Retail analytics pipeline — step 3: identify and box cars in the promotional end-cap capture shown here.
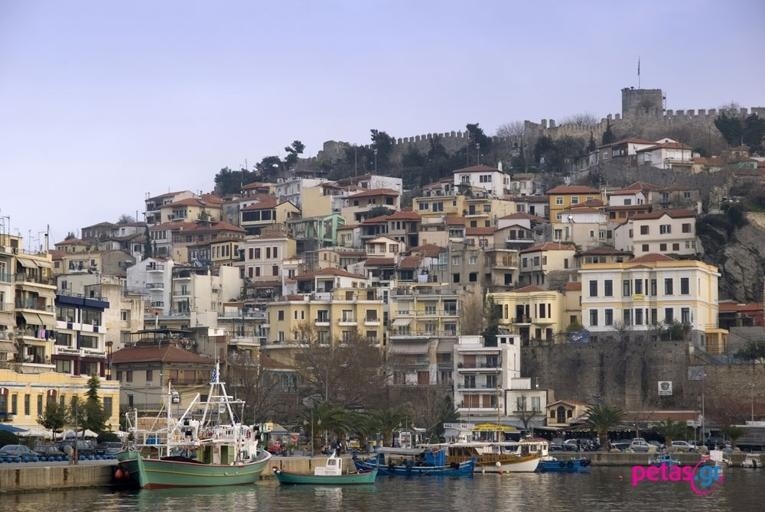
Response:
[1,442,125,457]
[550,438,765,453]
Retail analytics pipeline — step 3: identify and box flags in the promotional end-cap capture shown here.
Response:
[209,365,220,382]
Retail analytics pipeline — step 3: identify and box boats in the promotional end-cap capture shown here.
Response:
[116,362,272,491]
[377,440,591,474]
[352,448,475,480]
[274,458,377,484]
